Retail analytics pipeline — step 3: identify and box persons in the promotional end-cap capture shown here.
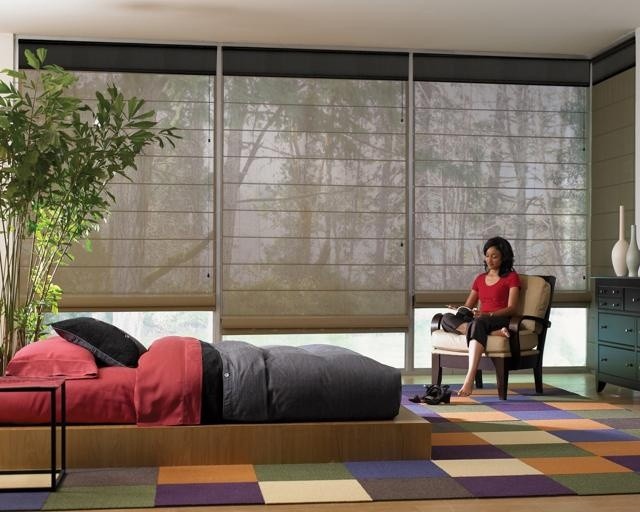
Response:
[458,236,522,398]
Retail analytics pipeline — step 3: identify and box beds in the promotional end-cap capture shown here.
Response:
[0,334,431,470]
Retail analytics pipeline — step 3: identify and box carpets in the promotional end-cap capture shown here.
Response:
[2,382,640,512]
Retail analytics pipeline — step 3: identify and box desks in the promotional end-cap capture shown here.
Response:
[1,376,66,492]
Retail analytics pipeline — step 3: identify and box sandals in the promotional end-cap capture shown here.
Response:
[408,384,452,405]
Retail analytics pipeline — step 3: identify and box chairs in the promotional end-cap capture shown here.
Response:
[431,275,556,400]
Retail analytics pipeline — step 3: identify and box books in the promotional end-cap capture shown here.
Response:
[444,305,474,322]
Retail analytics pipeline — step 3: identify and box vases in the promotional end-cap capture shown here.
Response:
[611,206,640,277]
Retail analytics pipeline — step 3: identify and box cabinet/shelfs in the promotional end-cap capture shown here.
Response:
[590,277,640,393]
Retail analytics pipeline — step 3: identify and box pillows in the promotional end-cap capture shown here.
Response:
[5,318,147,380]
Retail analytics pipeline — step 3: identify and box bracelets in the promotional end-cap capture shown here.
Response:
[489,312,494,318]
